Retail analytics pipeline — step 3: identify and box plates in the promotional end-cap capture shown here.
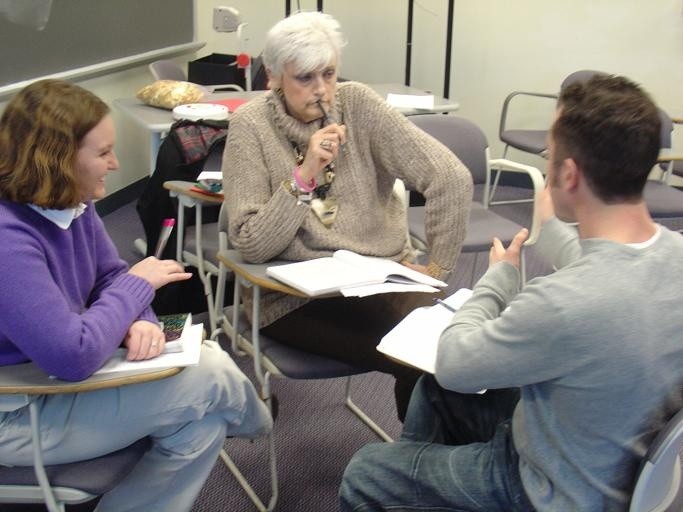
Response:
[172,103,229,122]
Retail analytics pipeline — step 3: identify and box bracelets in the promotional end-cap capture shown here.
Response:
[293,164,316,194]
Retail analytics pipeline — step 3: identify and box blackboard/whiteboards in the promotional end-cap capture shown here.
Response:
[0,0,205,99]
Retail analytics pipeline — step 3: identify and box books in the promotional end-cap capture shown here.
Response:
[266,250,450,298]
[47,313,203,377]
[377,288,489,397]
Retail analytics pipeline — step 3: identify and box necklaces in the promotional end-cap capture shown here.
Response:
[264,83,347,229]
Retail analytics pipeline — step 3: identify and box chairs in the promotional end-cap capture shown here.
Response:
[252,51,271,90]
[490,71,683,234]
[150,60,244,94]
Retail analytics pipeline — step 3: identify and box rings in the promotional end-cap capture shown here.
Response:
[149,341,158,347]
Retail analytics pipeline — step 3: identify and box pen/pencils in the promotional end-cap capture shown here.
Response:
[318,101,330,124]
[433,297,457,313]
[153,218,175,259]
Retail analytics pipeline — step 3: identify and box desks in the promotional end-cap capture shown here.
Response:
[112,83,459,260]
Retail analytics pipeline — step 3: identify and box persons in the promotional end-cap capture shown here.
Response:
[336,73,683,512]
[0,81,275,512]
[217,10,474,426]
